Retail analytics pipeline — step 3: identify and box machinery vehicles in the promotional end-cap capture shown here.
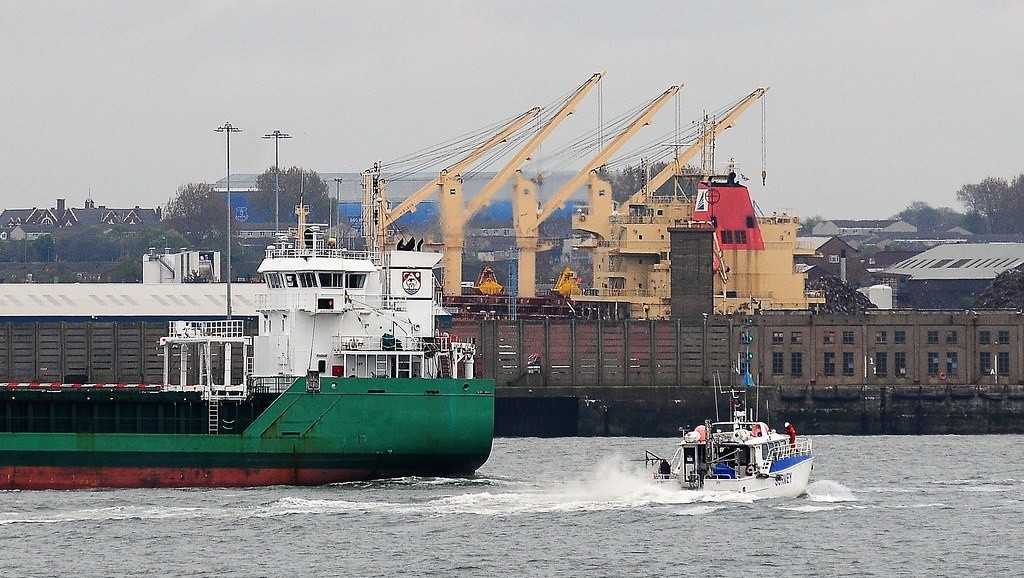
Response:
[362,69,769,302]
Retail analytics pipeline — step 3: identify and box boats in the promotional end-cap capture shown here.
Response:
[636,371,815,502]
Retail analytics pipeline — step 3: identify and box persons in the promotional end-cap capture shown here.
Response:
[784,422,796,448]
[659,460,671,479]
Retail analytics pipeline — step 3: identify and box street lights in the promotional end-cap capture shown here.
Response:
[334,178,344,247]
[261,130,293,248]
[213,122,242,322]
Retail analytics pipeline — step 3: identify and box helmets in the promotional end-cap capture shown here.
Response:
[784,422,790,428]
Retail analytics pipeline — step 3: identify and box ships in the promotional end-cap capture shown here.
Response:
[0,235,497,494]
